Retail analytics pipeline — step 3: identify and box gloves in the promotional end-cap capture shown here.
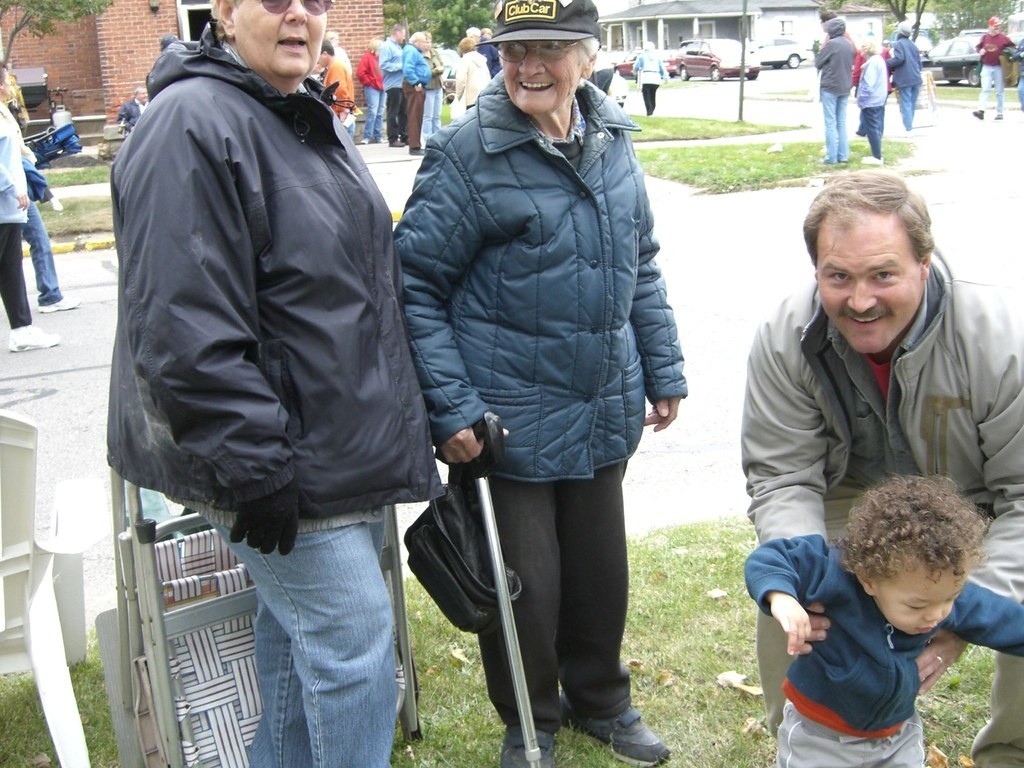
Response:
[229,471,300,557]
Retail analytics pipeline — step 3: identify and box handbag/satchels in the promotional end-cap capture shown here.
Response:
[404,482,522,633]
[441,88,467,126]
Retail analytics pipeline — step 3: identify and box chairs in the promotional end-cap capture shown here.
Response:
[0,412,93,768]
[98,437,422,768]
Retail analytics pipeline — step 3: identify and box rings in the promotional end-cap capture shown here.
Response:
[937,655,944,664]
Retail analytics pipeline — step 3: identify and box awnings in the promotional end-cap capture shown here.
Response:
[8,67,49,88]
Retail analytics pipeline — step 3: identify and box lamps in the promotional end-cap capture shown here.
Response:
[150,0,159,14]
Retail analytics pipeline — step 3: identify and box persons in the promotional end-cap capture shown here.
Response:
[852,27,893,137]
[886,24,922,138]
[819,10,858,63]
[854,41,888,165]
[735,171,1024,767]
[972,15,1014,121]
[311,24,624,154]
[1013,37,1024,113]
[744,471,1024,767]
[0,63,82,352]
[393,0,689,767]
[115,82,151,136]
[814,15,853,164]
[106,0,447,768]
[632,39,669,120]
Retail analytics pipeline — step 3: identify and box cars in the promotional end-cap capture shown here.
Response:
[675,36,760,82]
[751,37,809,70]
[885,28,1024,89]
[615,47,679,81]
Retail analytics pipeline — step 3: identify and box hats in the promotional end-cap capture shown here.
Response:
[475,0,601,60]
[989,16,1002,28]
[898,21,911,37]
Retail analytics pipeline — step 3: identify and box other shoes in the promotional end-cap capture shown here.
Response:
[856,131,867,136]
[360,138,370,144]
[861,155,884,165]
[819,157,848,166]
[376,139,388,144]
[389,141,405,147]
[975,742,1024,768]
[995,114,1003,120]
[973,109,984,120]
[50,197,63,211]
[409,146,425,155]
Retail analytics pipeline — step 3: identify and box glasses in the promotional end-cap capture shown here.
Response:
[493,39,584,62]
[261,0,335,16]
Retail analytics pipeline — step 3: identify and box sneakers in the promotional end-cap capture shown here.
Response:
[9,327,59,352]
[38,292,80,312]
[559,687,670,768]
[498,721,556,768]
